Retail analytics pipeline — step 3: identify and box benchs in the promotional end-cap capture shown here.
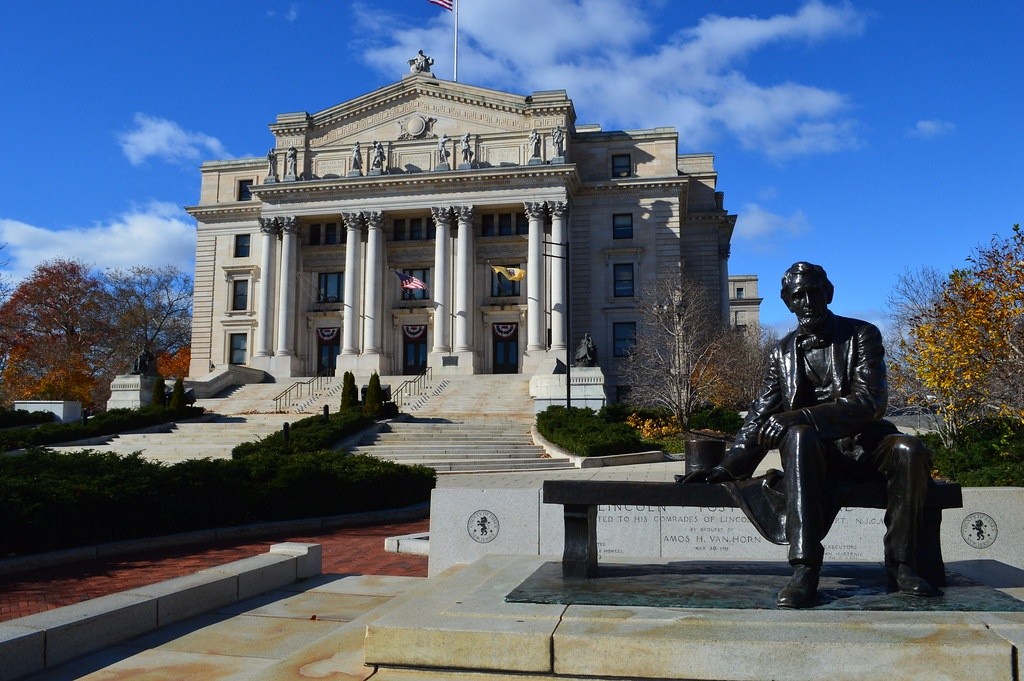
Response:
[543,479,963,586]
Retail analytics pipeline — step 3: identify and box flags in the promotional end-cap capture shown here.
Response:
[429,0,453,11]
[392,270,428,289]
[491,266,526,281]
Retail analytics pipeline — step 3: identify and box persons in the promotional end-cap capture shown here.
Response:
[406,49,430,73]
[129,347,156,376]
[677,263,936,607]
[577,332,593,360]
[265,125,565,177]
